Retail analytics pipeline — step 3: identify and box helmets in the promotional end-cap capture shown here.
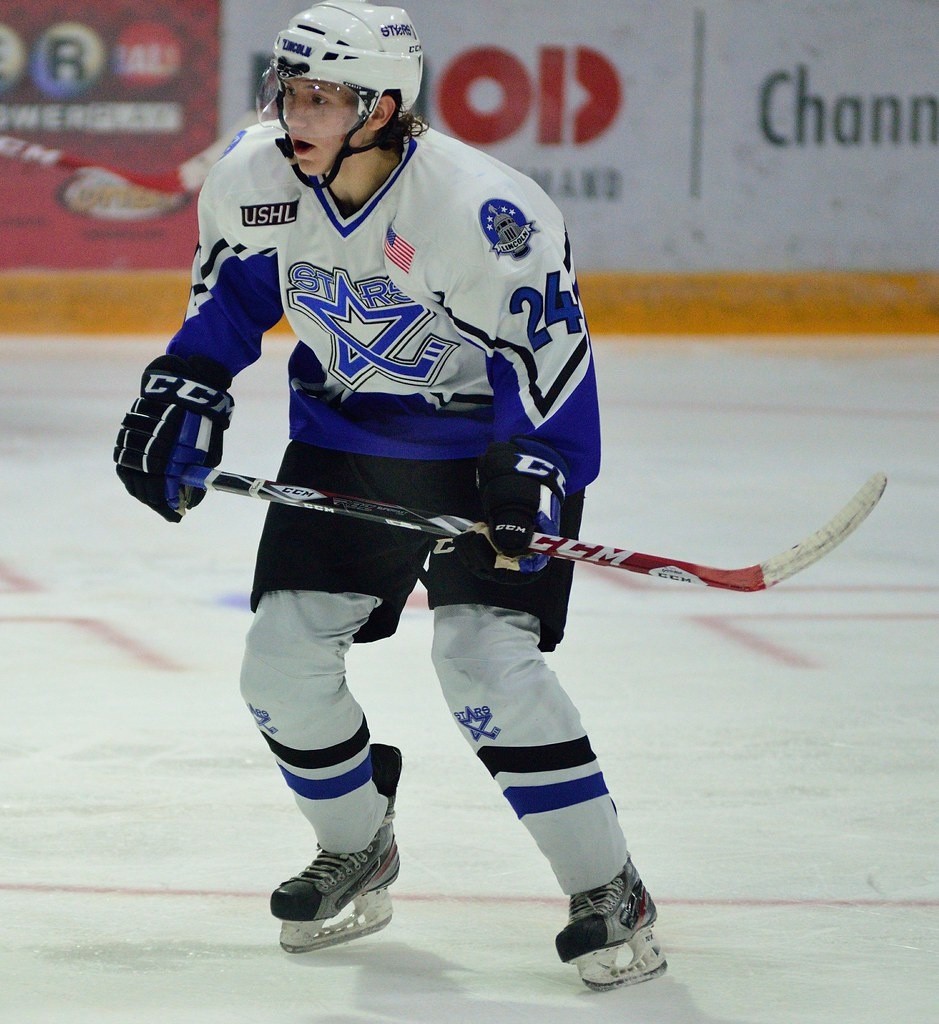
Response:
[272,0,424,123]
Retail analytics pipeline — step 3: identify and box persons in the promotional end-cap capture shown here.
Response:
[113,1,668,993]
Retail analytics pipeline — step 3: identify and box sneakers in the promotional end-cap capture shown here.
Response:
[269,791,403,954]
[555,851,667,993]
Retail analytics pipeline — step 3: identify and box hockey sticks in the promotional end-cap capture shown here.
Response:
[171,466,892,596]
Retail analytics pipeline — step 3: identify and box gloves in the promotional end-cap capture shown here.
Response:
[452,454,568,577]
[114,354,236,524]
